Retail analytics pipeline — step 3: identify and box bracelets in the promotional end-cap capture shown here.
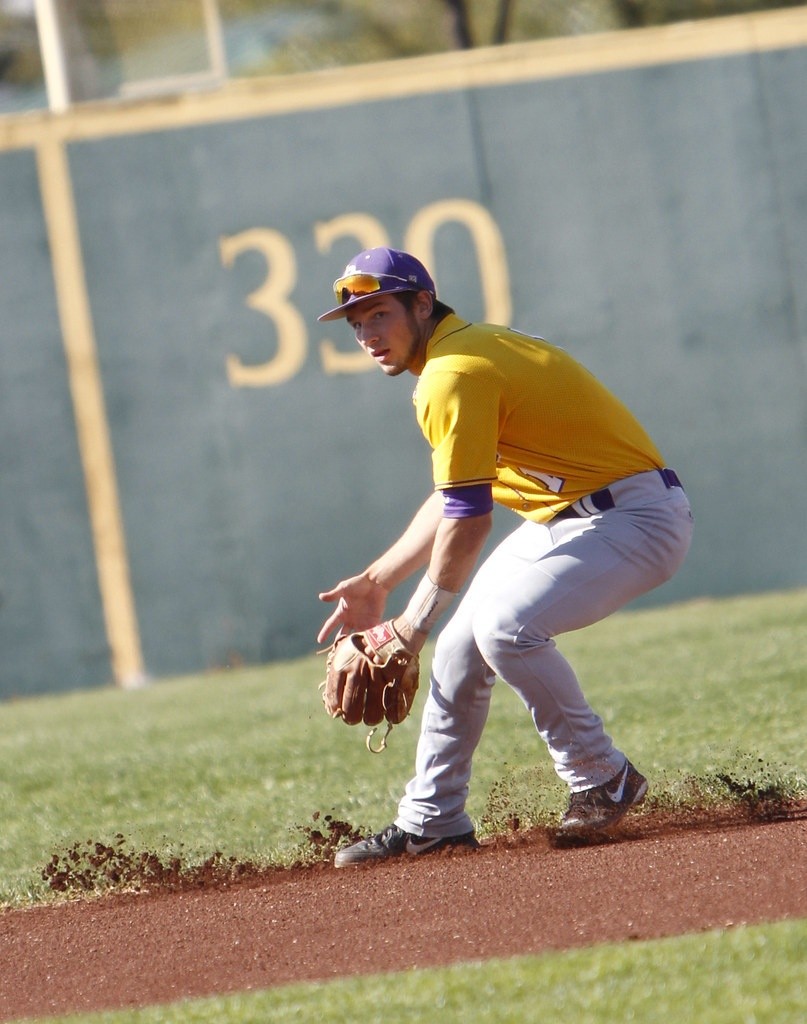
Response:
[403,571,461,634]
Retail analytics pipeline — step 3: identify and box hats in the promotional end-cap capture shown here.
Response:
[317,247,436,321]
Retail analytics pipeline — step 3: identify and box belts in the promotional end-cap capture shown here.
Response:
[555,469,682,518]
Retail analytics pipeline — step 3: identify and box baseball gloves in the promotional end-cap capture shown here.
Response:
[316,617,421,755]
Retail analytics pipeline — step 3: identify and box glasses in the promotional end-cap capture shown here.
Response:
[332,272,434,306]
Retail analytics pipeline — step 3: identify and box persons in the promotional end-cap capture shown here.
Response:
[317,247,694,870]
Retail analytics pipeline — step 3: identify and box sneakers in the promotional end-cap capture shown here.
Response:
[561,758,648,837]
[334,824,480,868]
